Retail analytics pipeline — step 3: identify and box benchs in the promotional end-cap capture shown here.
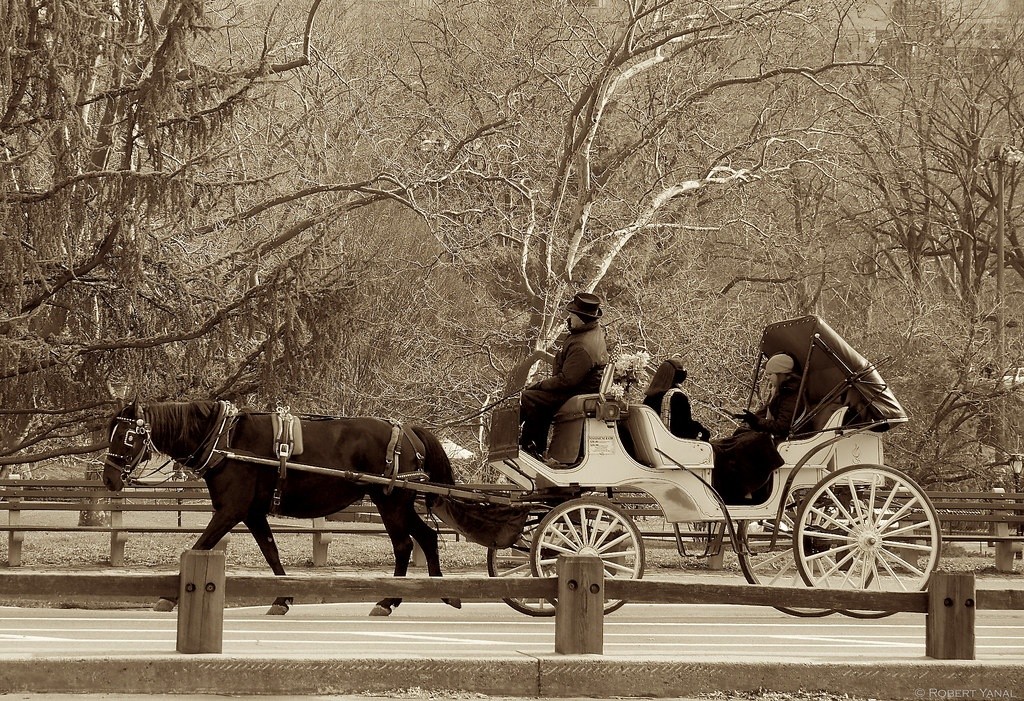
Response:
[628,402,714,469]
[777,402,849,470]
[546,341,620,463]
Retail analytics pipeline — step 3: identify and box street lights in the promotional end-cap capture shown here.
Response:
[974,144,1024,489]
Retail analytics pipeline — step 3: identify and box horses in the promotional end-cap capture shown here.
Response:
[102,393,462,616]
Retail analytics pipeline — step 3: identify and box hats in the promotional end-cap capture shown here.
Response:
[766,354,794,373]
[567,294,602,318]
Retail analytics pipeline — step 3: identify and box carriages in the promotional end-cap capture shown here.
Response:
[103,315,941,619]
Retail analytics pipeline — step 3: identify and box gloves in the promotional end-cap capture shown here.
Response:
[735,409,758,425]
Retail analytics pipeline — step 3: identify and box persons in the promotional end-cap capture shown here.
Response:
[643,360,709,440]
[731,352,809,498]
[517,291,609,460]
